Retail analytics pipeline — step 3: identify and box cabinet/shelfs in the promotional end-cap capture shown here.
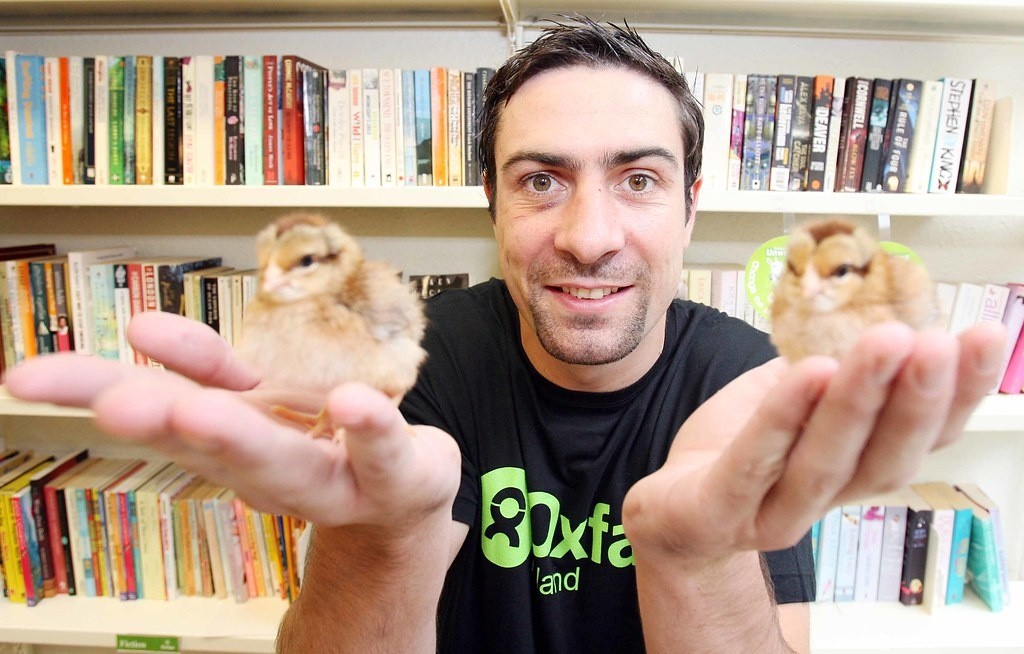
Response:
[0,0,1024,654]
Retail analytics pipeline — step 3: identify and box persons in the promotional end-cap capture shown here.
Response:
[0,21,1009,654]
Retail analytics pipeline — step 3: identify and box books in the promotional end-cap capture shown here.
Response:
[674,265,1024,394]
[0,448,313,607]
[0,50,497,188]
[0,243,470,384]
[664,57,1015,196]
[811,483,1010,612]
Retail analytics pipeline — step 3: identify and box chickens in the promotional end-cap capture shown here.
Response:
[767,220,946,369]
[232,211,428,446]
